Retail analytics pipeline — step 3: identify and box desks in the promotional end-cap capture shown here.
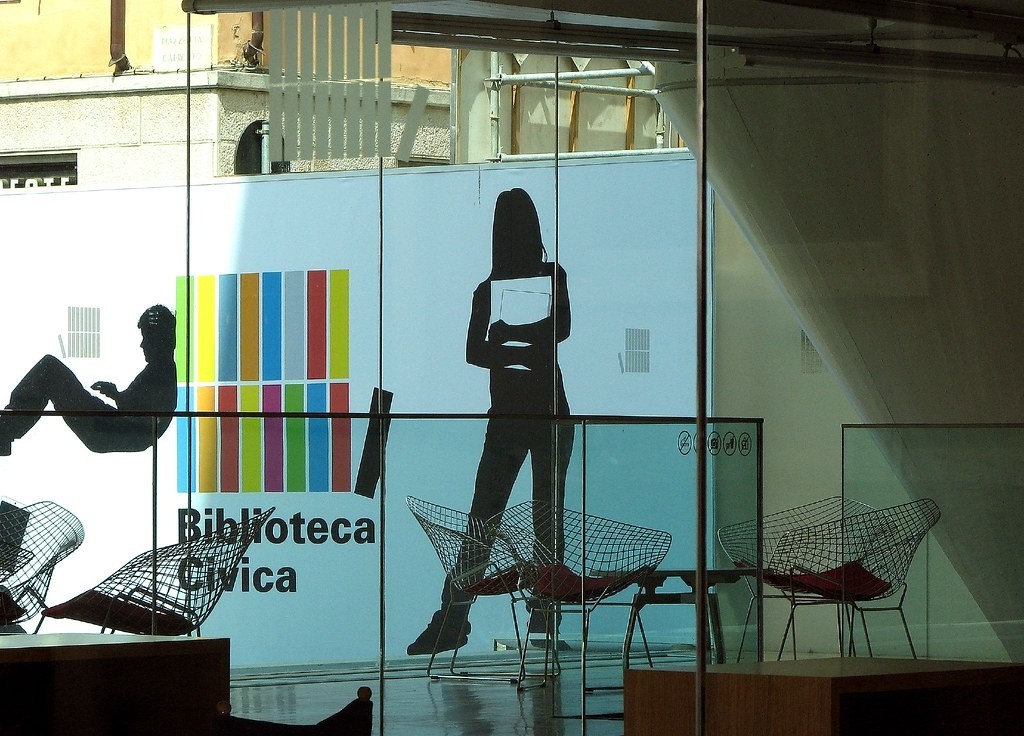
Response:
[589,568,772,671]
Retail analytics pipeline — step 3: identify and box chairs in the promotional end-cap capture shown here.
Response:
[41,506,276,637]
[405,494,563,684]
[0,500,86,635]
[716,493,942,664]
[483,499,672,692]
